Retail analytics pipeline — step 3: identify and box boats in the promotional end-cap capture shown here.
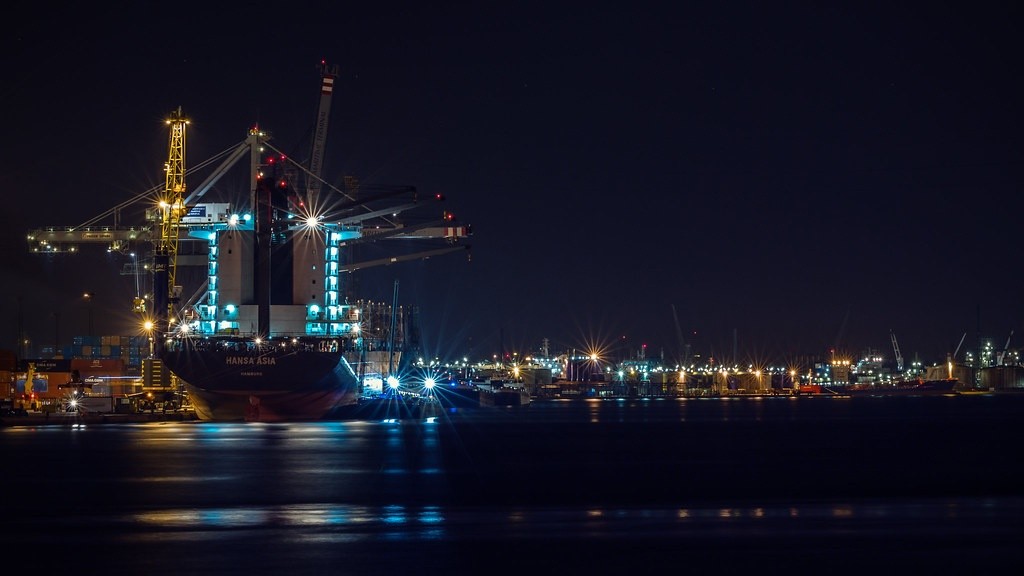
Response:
[496,388,531,413]
[825,377,958,398]
